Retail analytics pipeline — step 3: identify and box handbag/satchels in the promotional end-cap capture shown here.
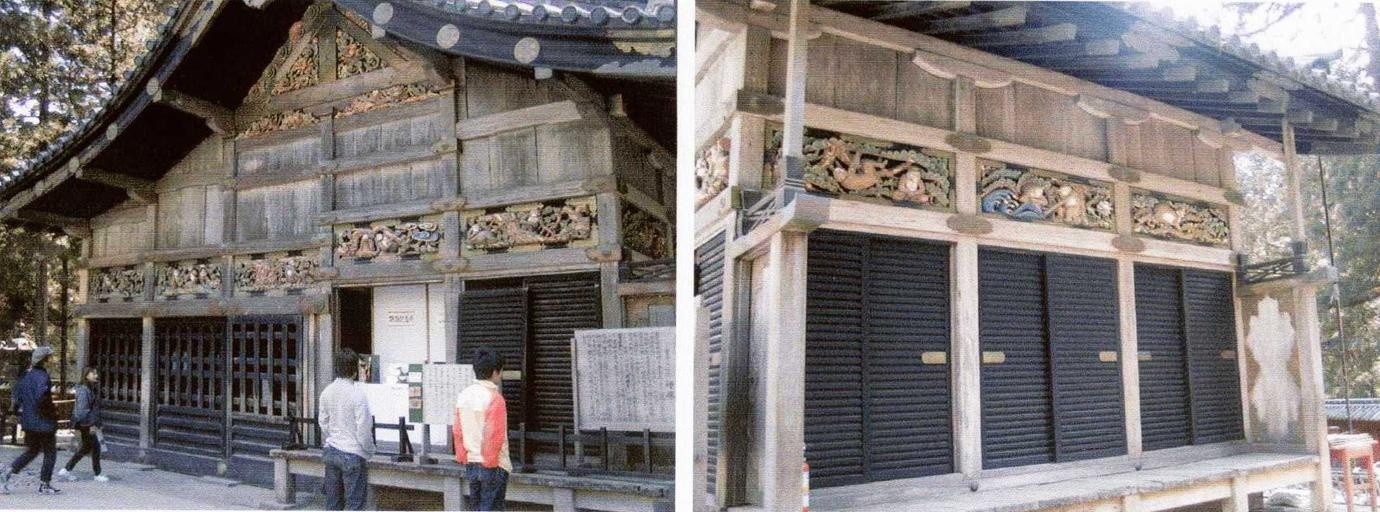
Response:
[70,415,80,429]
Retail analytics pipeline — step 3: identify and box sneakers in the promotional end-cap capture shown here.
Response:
[39,484,61,494]
[94,475,109,481]
[59,468,77,481]
[0,467,11,493]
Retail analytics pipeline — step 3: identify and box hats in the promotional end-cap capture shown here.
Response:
[32,348,51,364]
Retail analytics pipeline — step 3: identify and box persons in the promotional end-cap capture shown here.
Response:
[318,346,376,510]
[56,368,110,482]
[0,346,62,495]
[452,345,512,511]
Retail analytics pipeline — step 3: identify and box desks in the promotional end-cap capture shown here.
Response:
[1328,442,1378,512]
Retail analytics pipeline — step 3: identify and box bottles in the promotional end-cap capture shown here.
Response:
[96,429,108,453]
